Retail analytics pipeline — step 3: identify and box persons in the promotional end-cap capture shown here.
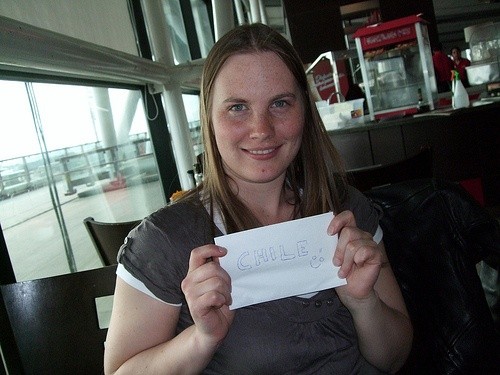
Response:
[104,23,413,375]
[432,46,471,94]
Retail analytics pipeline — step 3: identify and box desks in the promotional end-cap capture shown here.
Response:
[327,97,500,167]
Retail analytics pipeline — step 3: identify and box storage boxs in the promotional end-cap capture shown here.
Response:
[315,98,366,132]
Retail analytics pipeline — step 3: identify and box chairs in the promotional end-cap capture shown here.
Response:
[0,264,118,375]
[83,217,141,266]
[340,143,434,192]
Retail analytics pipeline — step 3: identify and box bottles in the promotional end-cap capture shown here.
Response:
[417,88,425,113]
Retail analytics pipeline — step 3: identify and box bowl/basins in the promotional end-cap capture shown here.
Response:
[466,61,499,86]
